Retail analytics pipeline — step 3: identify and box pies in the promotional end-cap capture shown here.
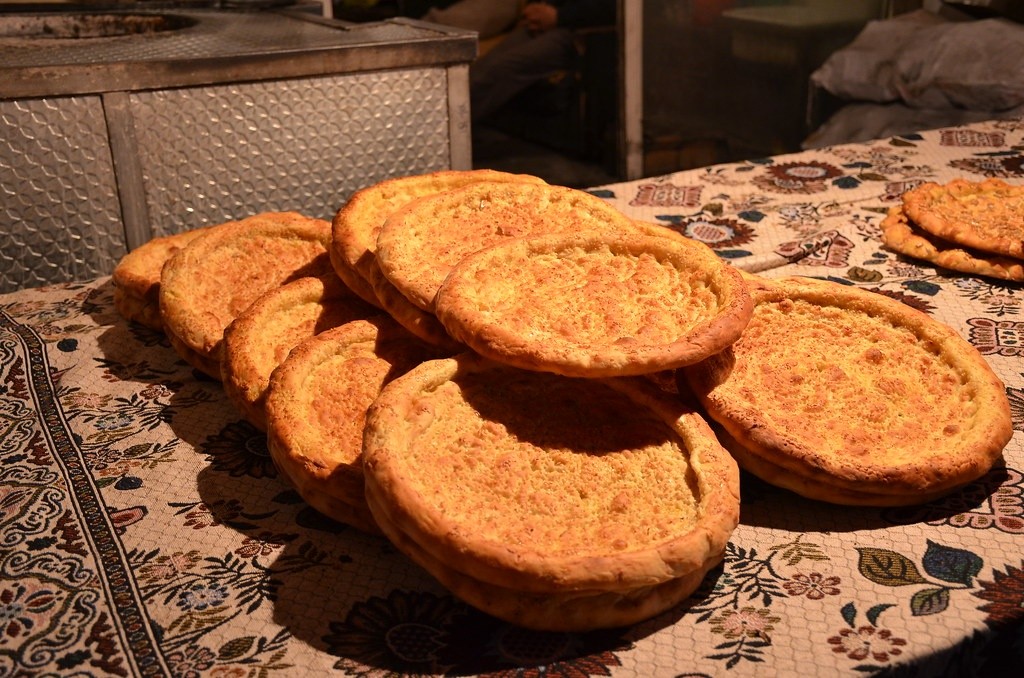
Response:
[111,169,1024,636]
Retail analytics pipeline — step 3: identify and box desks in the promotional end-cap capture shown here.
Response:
[0,117,1024,678]
